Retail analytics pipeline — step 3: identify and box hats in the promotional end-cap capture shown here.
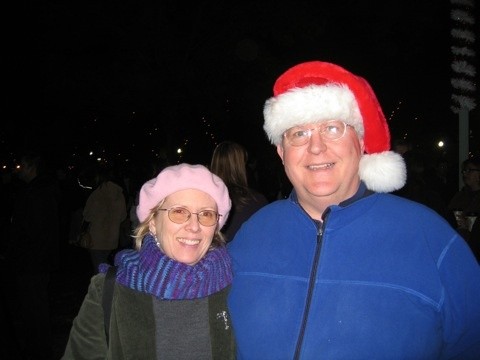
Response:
[135,163,232,231]
[263,61,406,193]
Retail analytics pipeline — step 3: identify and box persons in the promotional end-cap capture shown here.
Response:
[227,61,480,360]
[62,164,238,360]
[0,135,480,360]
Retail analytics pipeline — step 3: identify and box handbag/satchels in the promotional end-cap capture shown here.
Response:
[76,217,92,248]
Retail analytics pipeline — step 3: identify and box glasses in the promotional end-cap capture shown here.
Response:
[158,206,221,227]
[462,168,480,175]
[282,120,354,147]
